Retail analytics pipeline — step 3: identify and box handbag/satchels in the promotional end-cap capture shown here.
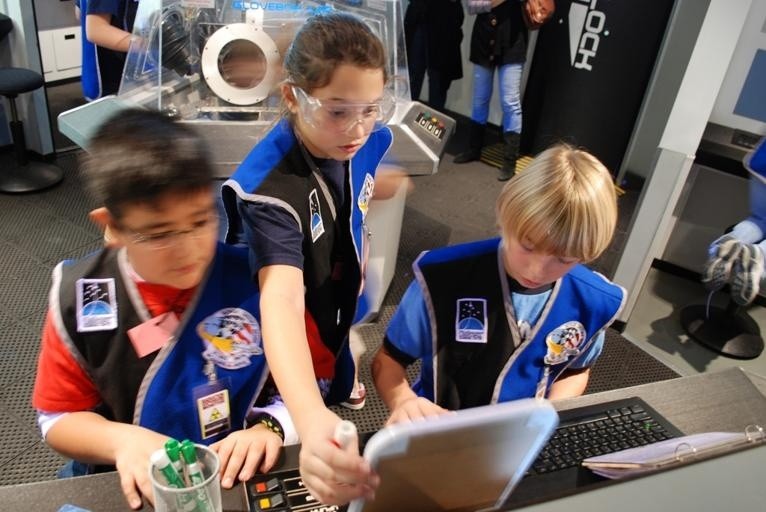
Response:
[521,0,556,29]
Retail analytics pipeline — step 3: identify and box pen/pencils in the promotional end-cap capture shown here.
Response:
[150,438,217,512]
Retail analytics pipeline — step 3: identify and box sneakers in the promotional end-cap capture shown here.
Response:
[701,234,762,307]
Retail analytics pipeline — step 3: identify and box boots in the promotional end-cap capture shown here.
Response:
[453,121,487,162]
[498,131,521,182]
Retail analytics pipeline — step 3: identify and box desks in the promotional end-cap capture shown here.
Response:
[1,367,766,512]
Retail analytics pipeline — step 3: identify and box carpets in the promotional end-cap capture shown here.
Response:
[480,133,629,199]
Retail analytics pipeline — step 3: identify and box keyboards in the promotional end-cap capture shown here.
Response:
[500,397,687,512]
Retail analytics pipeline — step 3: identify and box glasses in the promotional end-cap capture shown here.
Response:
[114,213,220,250]
[292,86,398,136]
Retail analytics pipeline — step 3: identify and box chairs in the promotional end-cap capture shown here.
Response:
[0,12,68,193]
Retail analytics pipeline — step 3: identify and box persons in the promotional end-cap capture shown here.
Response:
[370,143,631,429]
[223,11,393,509]
[403,1,467,112]
[452,2,532,179]
[702,140,766,304]
[75,1,153,102]
[31,107,338,512]
